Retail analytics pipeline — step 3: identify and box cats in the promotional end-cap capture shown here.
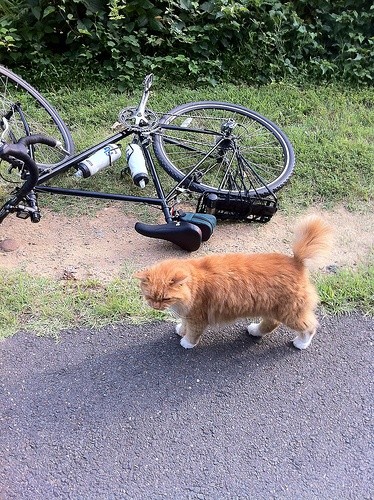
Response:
[133,215,330,350]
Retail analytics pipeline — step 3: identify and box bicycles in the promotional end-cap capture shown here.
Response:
[1,68,295,251]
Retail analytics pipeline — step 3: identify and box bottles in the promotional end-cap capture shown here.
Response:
[126,143,150,191]
[75,143,123,180]
